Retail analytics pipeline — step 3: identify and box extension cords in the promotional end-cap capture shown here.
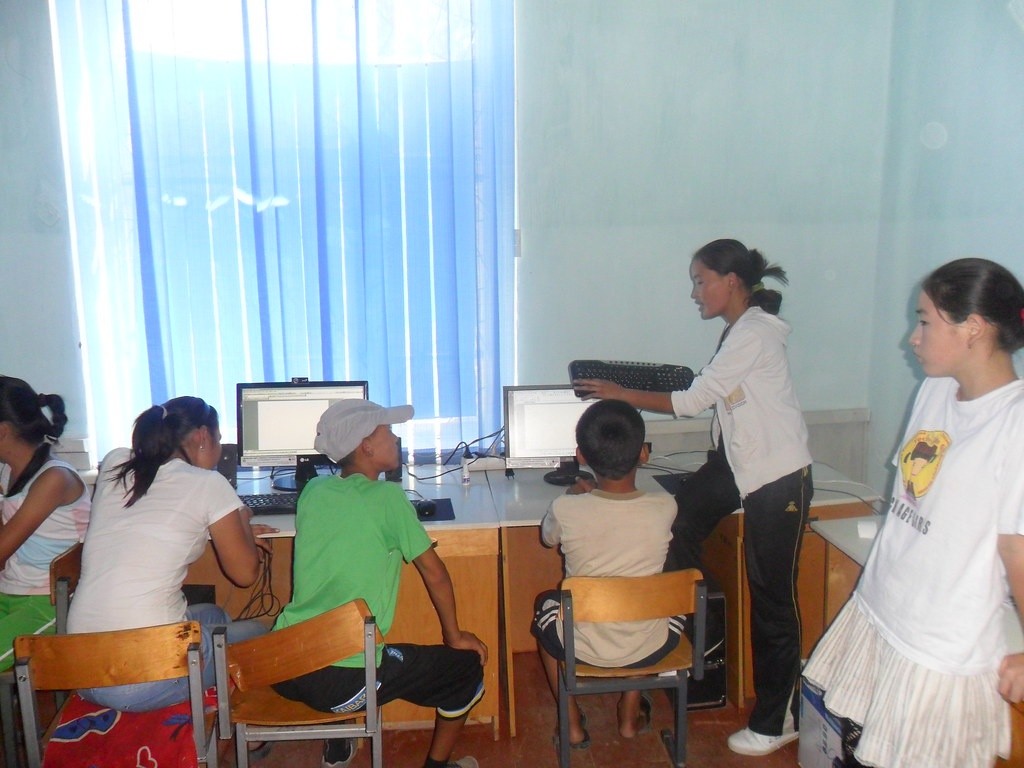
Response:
[460,455,506,471]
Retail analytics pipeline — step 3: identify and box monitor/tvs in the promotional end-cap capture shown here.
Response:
[236,380,369,491]
[503,384,594,485]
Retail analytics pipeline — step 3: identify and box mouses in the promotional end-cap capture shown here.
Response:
[417,500,436,518]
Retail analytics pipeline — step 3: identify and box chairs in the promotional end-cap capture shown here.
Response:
[555,568,707,768]
[0,540,383,768]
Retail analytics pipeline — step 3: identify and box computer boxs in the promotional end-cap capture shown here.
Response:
[670,579,728,709]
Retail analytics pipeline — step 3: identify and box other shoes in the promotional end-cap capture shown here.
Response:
[447,755,479,768]
[321,737,359,768]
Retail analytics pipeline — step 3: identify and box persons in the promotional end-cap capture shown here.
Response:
[0,374,92,673]
[801,258,1024,768]
[269,398,489,768]
[572,238,815,756]
[65,396,280,759]
[529,400,688,751]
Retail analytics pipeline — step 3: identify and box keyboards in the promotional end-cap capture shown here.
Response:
[238,493,299,514]
[569,359,694,398]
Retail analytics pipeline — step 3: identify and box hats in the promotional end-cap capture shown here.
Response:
[314,398,414,463]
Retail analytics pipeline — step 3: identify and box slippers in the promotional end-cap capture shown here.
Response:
[637,693,654,737]
[569,701,592,751]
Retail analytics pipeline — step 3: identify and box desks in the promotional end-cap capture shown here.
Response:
[811,514,1024,768]
[484,454,882,740]
[182,464,500,742]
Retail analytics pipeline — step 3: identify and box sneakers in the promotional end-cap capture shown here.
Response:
[726,714,799,756]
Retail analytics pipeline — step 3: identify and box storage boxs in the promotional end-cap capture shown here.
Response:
[798,659,842,768]
